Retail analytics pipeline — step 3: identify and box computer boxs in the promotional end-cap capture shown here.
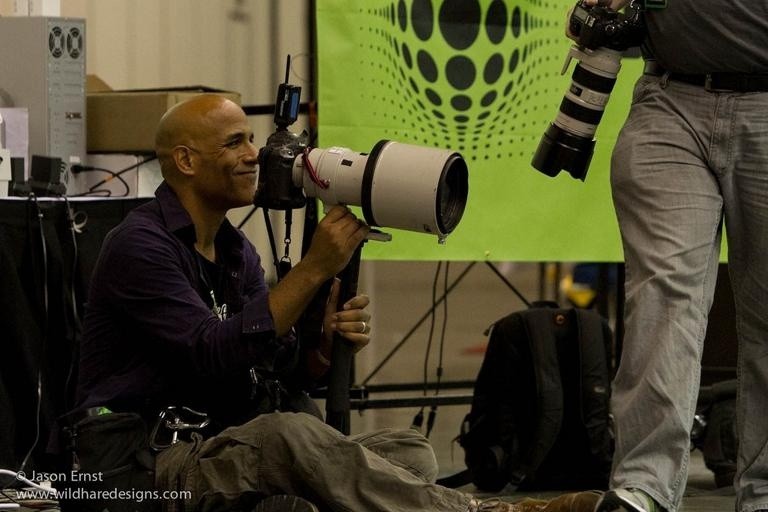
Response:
[0,16,88,197]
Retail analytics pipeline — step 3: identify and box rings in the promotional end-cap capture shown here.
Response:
[360,321,366,333]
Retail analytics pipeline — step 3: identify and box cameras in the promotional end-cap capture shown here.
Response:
[531,0,642,183]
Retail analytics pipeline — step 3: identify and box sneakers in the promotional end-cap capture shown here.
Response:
[252,495,318,512]
[594,487,668,512]
[466,490,603,512]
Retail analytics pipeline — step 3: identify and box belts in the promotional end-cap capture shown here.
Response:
[643,58,768,93]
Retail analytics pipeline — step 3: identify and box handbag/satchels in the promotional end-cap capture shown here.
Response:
[62,413,148,478]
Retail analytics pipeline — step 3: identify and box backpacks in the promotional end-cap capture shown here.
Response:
[460,301,615,492]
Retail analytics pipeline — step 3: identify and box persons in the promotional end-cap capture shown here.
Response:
[67,93,619,512]
[576,1,768,512]
